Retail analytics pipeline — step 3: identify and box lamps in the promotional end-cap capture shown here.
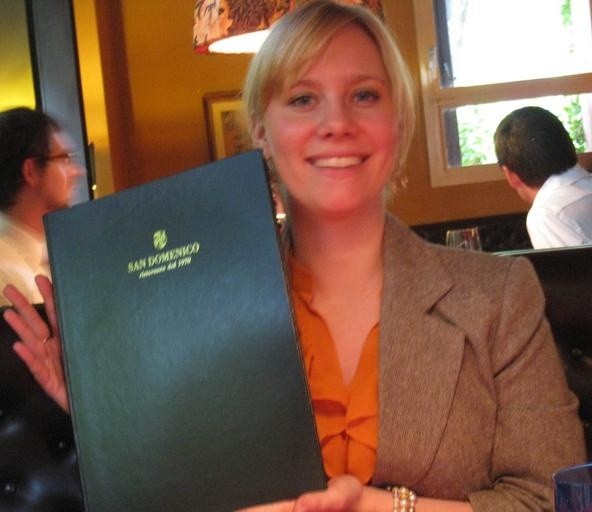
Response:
[192,1,384,57]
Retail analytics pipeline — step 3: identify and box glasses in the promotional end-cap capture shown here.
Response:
[49,150,73,161]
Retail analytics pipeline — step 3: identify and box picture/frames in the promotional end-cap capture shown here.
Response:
[202,89,288,225]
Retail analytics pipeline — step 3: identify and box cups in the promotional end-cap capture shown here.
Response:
[552,462,592,511]
[444,229,481,251]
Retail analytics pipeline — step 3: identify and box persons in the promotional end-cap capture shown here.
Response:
[493,104,591,250]
[0,106,82,306]
[3,0,588,512]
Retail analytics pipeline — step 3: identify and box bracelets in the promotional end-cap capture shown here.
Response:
[387,482,418,512]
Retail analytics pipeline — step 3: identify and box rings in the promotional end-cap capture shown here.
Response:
[42,335,52,343]
